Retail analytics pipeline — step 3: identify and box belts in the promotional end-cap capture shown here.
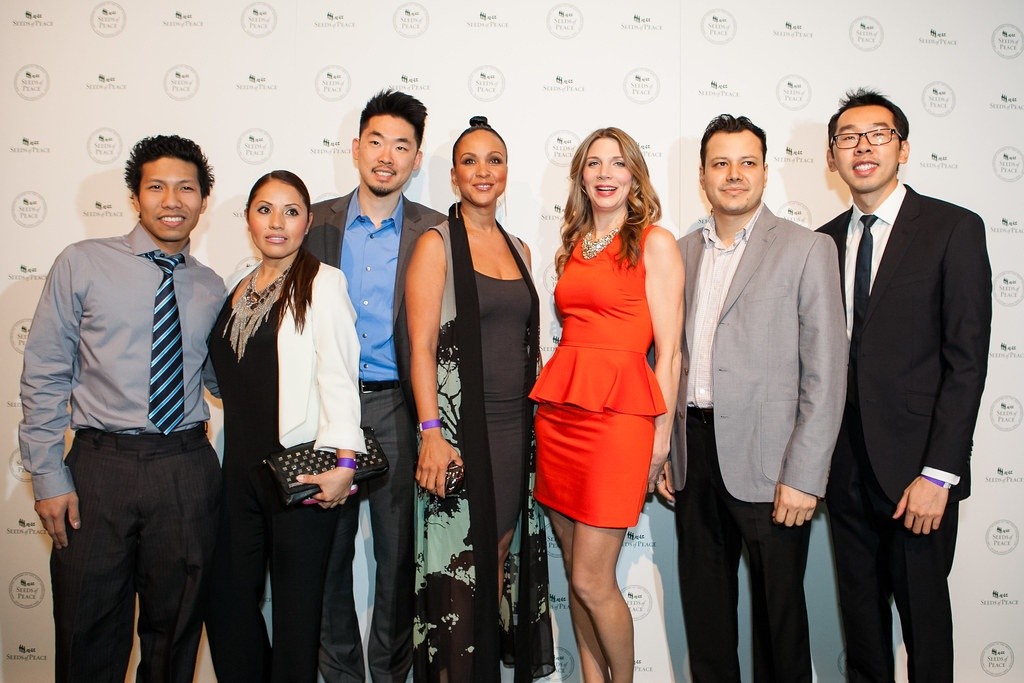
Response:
[687,408,714,424]
[359,380,400,394]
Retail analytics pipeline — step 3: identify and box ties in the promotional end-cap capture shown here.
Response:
[848,214,877,398]
[138,251,185,436]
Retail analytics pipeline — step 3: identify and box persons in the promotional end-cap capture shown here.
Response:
[403,116,545,683]
[811,88,992,683]
[18,134,229,683]
[528,127,685,683]
[646,113,849,683]
[204,170,368,683]
[306,88,449,683]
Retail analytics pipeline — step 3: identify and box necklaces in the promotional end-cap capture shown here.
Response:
[581,227,620,261]
[222,263,294,364]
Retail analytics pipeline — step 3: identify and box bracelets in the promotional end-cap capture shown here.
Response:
[418,419,442,432]
[920,475,951,489]
[336,458,359,470]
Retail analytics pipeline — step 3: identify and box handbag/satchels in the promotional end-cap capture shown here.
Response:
[261,426,388,505]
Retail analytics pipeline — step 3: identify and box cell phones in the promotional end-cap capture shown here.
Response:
[301,483,359,504]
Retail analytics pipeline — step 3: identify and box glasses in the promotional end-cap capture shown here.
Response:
[829,128,905,150]
